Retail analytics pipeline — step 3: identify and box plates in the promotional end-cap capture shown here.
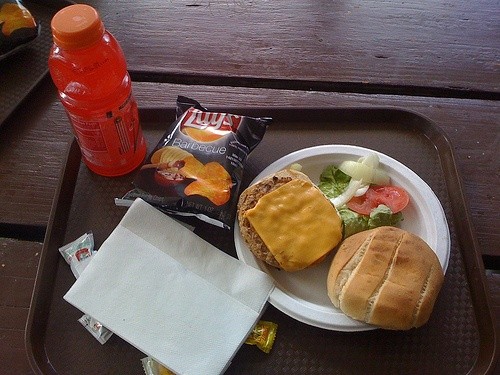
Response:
[234,144,451,332]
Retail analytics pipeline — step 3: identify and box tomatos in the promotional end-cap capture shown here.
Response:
[346,183,409,216]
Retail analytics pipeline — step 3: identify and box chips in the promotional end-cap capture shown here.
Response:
[0,2,37,36]
[150,146,233,206]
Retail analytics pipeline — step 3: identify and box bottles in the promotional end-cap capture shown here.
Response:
[48,4,148,179]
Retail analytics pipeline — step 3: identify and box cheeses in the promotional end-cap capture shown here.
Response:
[244,180,343,271]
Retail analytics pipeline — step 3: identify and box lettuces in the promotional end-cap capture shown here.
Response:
[317,163,404,239]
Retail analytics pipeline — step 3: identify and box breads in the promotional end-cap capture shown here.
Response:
[327,226,445,330]
[236,169,344,272]
